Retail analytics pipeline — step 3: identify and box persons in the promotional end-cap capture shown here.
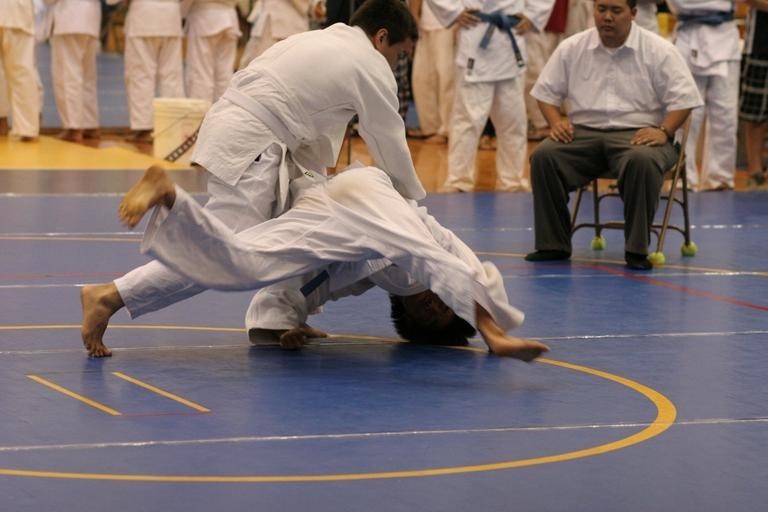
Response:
[524,0,705,271]
[78,1,429,356]
[119,162,548,361]
[0,1,746,194]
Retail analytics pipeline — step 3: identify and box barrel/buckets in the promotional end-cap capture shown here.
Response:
[151,97,212,164]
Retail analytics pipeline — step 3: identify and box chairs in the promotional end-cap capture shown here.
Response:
[570,108,698,268]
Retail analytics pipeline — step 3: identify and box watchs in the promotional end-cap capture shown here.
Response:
[655,127,671,138]
